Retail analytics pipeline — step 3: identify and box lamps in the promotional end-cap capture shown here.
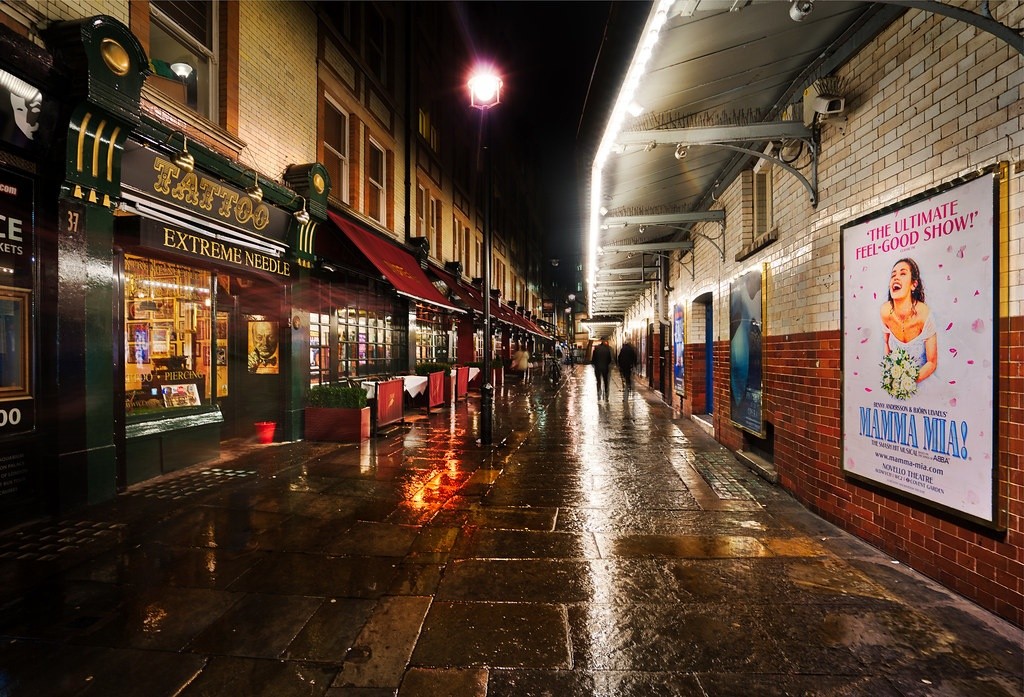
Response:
[164,130,194,172]
[286,195,309,224]
[240,168,263,202]
[170,63,193,104]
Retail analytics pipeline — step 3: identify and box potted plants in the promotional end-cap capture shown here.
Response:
[415,362,455,407]
[303,384,371,443]
[464,359,505,387]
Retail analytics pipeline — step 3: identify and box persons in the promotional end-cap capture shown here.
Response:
[618,341,636,391]
[514,345,529,386]
[252,322,279,359]
[592,342,612,394]
[880,258,938,400]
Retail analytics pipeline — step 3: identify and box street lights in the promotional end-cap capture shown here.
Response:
[564,293,575,373]
[468,61,503,447]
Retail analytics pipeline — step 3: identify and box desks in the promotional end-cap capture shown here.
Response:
[392,376,428,410]
[468,366,480,382]
[362,380,378,400]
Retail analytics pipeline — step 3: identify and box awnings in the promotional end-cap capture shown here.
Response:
[429,266,553,341]
[329,212,467,315]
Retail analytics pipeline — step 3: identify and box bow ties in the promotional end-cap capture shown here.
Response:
[263,356,277,367]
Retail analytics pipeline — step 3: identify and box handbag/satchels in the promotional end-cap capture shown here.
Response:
[511,360,518,368]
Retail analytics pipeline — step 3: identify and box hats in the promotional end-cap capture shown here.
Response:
[600,335,610,340]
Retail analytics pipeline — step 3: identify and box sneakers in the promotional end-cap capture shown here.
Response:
[605,402,609,404]
[598,400,602,404]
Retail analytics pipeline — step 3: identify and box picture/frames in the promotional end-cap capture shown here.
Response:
[126,272,227,369]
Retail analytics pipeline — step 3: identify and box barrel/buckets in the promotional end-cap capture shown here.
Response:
[254,421,277,444]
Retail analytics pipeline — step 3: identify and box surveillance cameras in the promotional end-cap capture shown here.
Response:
[753,141,783,174]
[813,94,845,113]
